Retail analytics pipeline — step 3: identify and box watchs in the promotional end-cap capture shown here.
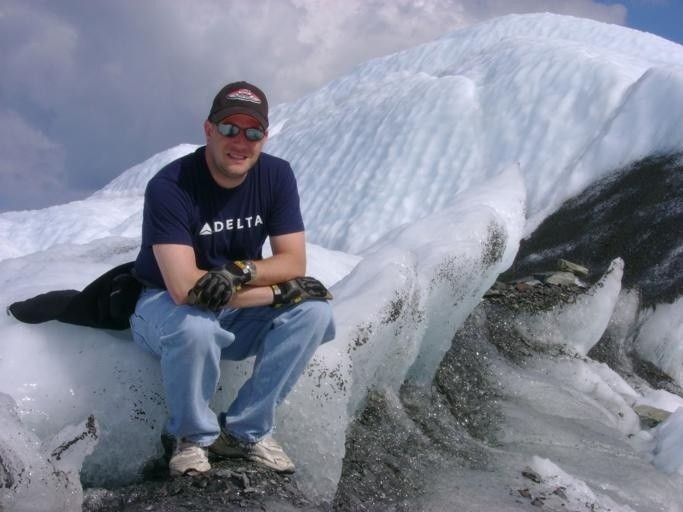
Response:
[241,258,257,286]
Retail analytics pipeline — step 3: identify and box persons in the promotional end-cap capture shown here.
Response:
[129,81,334,478]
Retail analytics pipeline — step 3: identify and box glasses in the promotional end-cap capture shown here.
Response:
[214,123,266,141]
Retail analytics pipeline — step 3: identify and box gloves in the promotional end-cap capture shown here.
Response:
[188,259,255,311]
[271,277,333,309]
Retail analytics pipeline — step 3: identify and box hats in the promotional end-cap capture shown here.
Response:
[209,82,269,129]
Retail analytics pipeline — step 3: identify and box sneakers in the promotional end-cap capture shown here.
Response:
[161,428,211,479]
[209,412,295,474]
